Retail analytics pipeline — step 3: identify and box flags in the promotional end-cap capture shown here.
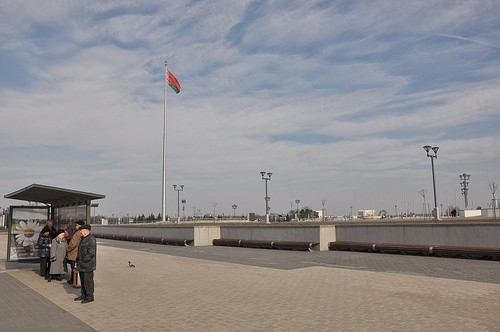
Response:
[163,66,182,94]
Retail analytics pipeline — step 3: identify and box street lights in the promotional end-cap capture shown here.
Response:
[424,146,438,219]
[295,199,300,224]
[173,184,185,225]
[459,173,470,209]
[182,200,187,222]
[232,204,237,219]
[90,203,99,224]
[260,172,272,222]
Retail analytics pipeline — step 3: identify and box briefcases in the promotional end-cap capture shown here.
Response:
[73,270,81,287]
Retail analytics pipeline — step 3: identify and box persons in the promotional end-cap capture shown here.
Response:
[451,209,456,217]
[47,229,68,282]
[74,223,96,304]
[37,229,53,279]
[67,221,86,284]
[41,220,56,236]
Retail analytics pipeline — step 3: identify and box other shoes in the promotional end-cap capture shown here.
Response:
[42,274,62,282]
[81,299,90,303]
[67,278,73,284]
[74,297,83,300]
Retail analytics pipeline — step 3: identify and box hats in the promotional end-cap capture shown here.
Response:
[79,224,91,230]
[56,229,64,236]
[42,225,50,233]
[75,220,85,225]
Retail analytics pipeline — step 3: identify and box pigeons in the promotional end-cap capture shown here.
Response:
[128,260,135,267]
